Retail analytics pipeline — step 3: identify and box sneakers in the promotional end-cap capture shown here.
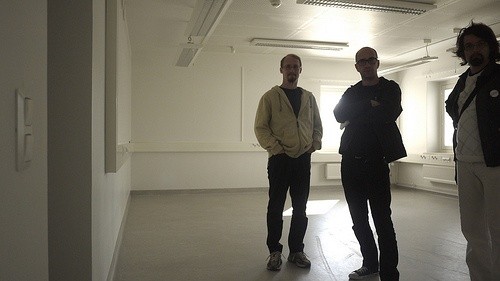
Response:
[288,251,311,268]
[348,266,379,280]
[265,251,285,271]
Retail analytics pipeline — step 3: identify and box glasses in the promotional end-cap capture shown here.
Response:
[463,43,489,51]
[357,58,377,64]
[283,65,302,69]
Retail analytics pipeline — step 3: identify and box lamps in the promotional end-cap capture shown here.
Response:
[377,38,439,78]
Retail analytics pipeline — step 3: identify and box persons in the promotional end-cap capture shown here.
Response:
[254,54,324,270]
[333,47,407,281]
[444,23,500,281]
